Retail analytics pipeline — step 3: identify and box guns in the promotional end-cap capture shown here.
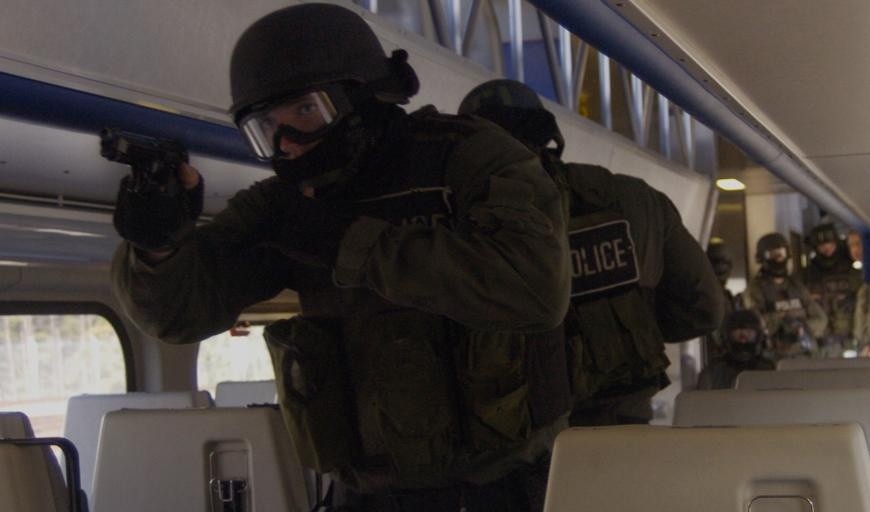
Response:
[99,124,188,194]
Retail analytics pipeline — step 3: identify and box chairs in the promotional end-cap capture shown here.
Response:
[670,384,870,430]
[734,368,868,386]
[88,402,320,512]
[776,358,867,371]
[212,380,282,408]
[543,421,868,511]
[2,410,92,510]
[58,389,211,511]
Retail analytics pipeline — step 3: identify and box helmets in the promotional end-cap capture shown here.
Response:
[224,3,410,122]
[718,312,762,343]
[708,236,734,284]
[456,78,556,120]
[756,232,791,266]
[809,223,839,250]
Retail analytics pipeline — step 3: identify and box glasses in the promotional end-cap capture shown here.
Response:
[236,85,353,164]
[763,245,789,265]
[810,229,835,245]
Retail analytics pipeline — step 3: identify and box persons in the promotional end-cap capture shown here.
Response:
[700,223,868,389]
[112,0,575,512]
[456,78,724,426]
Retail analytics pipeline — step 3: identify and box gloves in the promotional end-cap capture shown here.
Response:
[111,165,206,250]
[286,179,364,267]
